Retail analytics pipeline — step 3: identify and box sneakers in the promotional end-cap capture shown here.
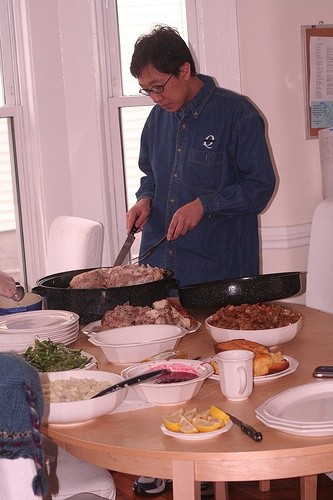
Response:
[132,475,173,495]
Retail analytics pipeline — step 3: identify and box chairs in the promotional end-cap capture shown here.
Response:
[45,216,104,276]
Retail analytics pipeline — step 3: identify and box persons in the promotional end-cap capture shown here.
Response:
[0,272,17,298]
[125,26,277,496]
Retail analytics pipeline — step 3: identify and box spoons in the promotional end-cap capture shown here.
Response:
[12,286,25,301]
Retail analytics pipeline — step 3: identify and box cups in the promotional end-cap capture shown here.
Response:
[215,349,256,402]
[0,292,43,316]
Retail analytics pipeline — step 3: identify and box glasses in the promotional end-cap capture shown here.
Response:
[139,73,174,96]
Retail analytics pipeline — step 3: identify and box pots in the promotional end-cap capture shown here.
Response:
[31,266,175,325]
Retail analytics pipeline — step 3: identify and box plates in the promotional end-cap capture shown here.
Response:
[17,347,98,370]
[160,420,233,440]
[200,354,299,384]
[254,378,333,437]
[81,320,202,337]
[0,310,79,353]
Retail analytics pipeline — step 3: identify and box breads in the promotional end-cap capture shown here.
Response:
[208,339,289,377]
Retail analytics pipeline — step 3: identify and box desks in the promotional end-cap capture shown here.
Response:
[36,296,333,500]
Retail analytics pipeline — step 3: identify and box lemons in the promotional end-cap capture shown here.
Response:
[163,405,231,434]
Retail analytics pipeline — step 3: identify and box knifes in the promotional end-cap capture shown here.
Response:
[225,410,263,442]
[113,215,139,267]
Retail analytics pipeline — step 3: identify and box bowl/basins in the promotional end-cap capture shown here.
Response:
[38,370,129,427]
[205,309,303,350]
[121,358,215,406]
[87,324,189,365]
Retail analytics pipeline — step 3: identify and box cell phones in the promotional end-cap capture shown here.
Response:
[314,366,333,378]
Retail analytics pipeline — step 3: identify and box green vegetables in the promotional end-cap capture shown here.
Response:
[16,336,94,372]
